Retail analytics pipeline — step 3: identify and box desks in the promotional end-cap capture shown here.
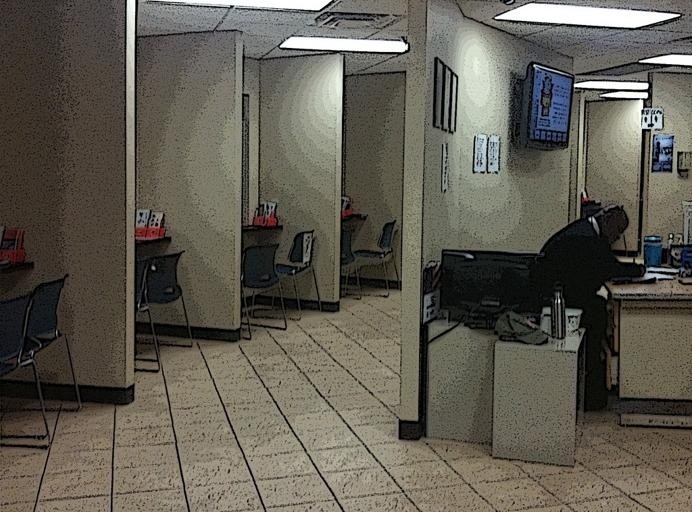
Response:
[604,255,692,401]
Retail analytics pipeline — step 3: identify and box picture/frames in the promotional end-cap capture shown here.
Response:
[487,134,501,174]
[652,133,674,173]
[432,56,459,133]
[473,133,487,173]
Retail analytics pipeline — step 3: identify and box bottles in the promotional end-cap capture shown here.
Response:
[644,235,663,268]
[552,287,566,340]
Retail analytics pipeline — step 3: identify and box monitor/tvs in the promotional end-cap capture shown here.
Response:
[520,61,575,151]
[439,248,545,328]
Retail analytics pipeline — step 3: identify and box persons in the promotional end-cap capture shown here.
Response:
[534,206,647,411]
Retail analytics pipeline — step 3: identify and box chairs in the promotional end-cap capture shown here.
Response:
[241,230,322,341]
[136,251,193,372]
[0,275,81,450]
[341,221,401,298]
